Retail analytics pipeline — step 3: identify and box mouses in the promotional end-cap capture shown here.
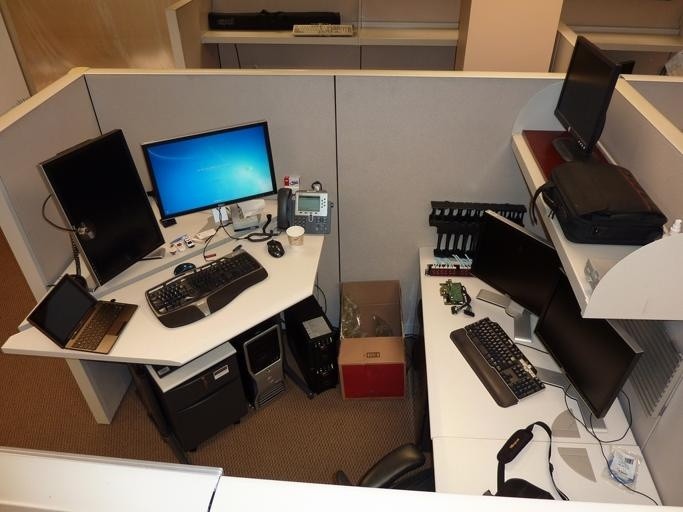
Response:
[267,239,285,257]
[174,262,194,275]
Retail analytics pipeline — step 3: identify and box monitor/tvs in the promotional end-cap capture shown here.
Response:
[470,209,563,343]
[534,268,643,432]
[554,34,621,162]
[35,128,167,292]
[140,120,278,241]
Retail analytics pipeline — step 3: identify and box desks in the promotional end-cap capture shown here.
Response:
[419,127,683,502]
[2,198,325,367]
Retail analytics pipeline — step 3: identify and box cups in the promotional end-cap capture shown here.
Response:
[286,226,306,249]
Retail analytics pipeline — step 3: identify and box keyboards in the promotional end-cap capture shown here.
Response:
[145,249,267,328]
[450,317,546,408]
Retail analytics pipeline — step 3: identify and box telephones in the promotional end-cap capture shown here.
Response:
[277,188,333,234]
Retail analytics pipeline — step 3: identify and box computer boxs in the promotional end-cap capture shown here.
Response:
[233,322,285,410]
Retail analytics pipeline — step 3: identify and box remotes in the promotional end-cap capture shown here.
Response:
[292,24,354,36]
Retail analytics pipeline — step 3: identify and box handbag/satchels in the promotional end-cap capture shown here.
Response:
[542,160,668,246]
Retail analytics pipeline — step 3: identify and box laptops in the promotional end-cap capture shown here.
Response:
[26,273,138,354]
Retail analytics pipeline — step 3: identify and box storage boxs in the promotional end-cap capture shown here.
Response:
[336,279,409,398]
